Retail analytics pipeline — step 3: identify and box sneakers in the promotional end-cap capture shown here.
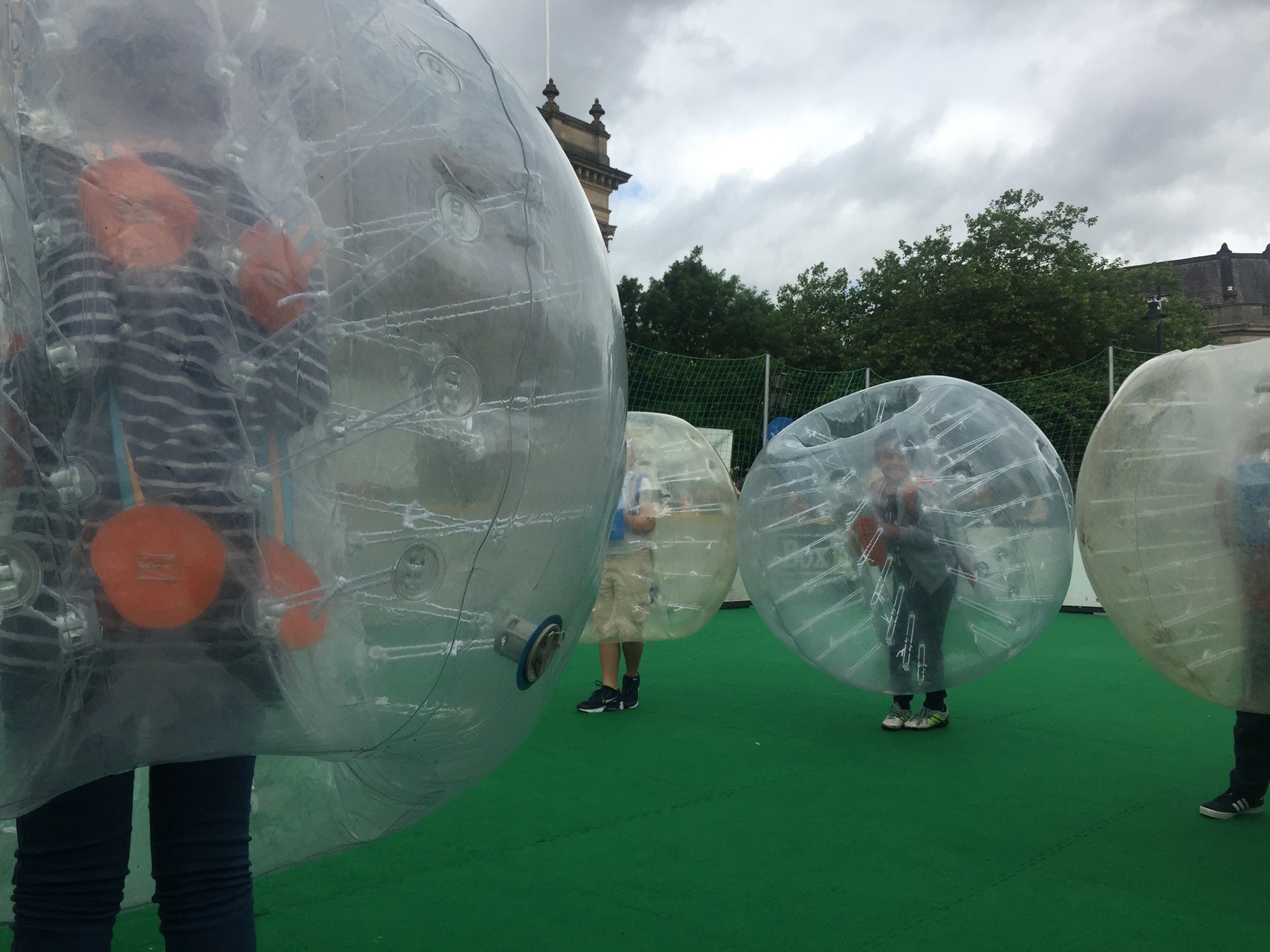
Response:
[577,680,621,713]
[620,671,640,709]
[1199,788,1264,820]
[882,703,911,729]
[903,703,949,730]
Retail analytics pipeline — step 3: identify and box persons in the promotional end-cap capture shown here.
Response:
[1198,381,1269,822]
[1,0,951,952]
